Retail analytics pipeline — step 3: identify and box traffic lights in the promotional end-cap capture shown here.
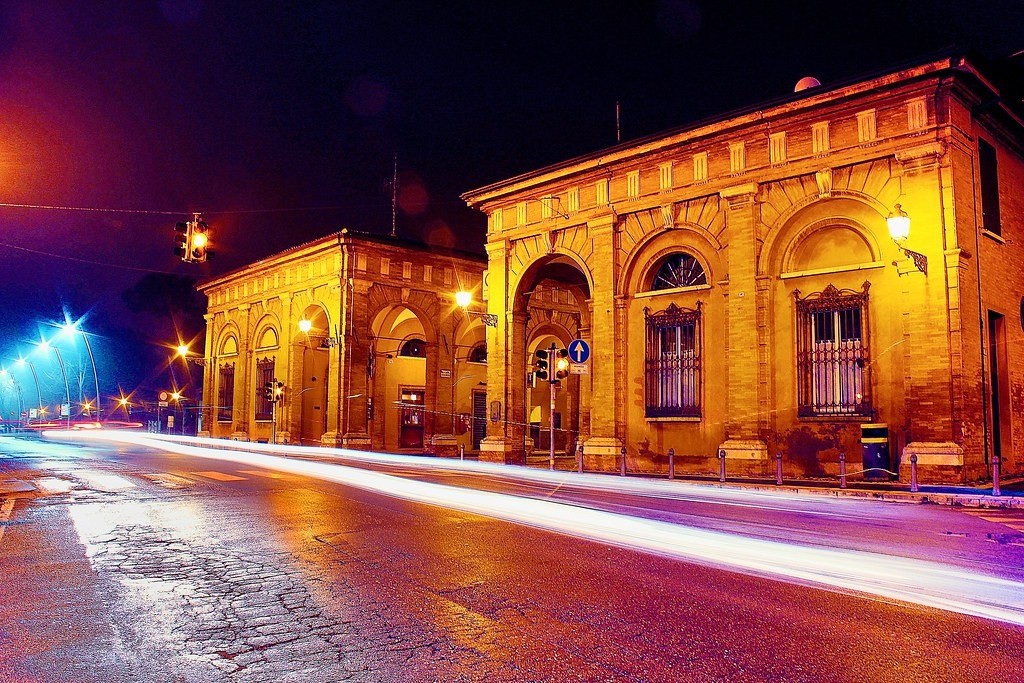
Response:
[174,219,214,265]
[264,380,284,402]
[536,346,570,380]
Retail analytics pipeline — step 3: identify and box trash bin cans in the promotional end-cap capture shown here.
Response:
[860,422,890,482]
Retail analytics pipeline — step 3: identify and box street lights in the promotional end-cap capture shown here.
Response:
[0,369,21,432]
[18,358,42,432]
[65,320,100,423]
[38,340,72,432]
